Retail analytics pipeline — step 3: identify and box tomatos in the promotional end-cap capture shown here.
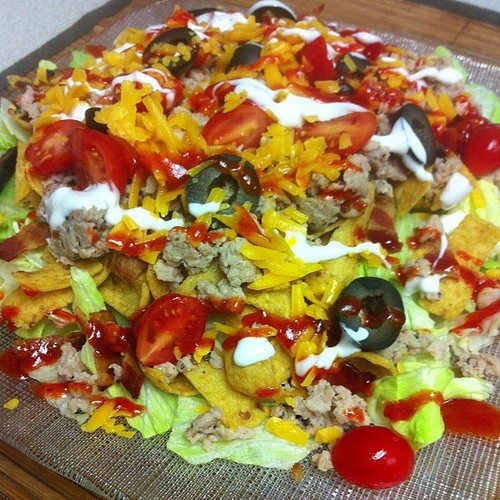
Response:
[26,104,500,491]
